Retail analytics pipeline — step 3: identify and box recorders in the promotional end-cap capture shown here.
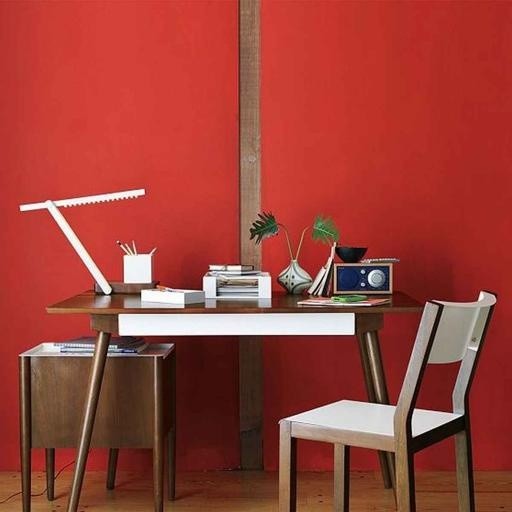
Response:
[333,262,394,296]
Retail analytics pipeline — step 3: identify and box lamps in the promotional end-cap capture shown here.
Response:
[18,188,160,294]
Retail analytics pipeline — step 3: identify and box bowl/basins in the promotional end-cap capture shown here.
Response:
[332,246,367,261]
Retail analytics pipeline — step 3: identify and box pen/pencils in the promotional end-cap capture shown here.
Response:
[150,248,157,254]
[156,285,178,292]
[117,239,137,255]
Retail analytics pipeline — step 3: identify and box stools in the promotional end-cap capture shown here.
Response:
[17,342,177,512]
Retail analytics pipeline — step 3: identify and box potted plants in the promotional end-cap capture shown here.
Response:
[249,212,339,293]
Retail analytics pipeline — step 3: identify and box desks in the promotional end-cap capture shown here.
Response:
[45,288,426,512]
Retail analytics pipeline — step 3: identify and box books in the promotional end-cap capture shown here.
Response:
[207,263,261,295]
[296,296,392,307]
[359,256,399,265]
[53,335,151,355]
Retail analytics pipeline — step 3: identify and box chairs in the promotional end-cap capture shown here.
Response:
[277,292,497,512]
